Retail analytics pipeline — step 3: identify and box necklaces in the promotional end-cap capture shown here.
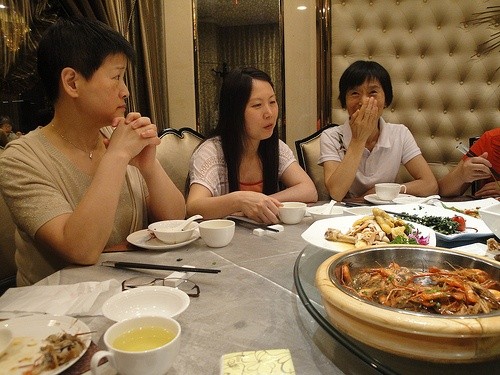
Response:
[52,124,100,159]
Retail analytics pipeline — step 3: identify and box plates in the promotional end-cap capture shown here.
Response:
[443,197,500,215]
[0,314,92,375]
[299,215,437,254]
[364,193,415,204]
[344,203,494,243]
[126,229,199,250]
[392,197,440,204]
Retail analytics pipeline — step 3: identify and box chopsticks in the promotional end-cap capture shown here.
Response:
[227,217,279,233]
[455,141,500,179]
[102,260,222,274]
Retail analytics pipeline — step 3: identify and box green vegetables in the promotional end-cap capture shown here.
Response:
[387,223,416,244]
[396,205,460,235]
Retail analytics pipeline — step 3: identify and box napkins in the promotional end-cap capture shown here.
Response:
[0,278,122,321]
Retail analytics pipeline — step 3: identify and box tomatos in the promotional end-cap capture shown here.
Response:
[452,216,466,231]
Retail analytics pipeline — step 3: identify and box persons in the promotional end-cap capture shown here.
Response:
[439,128,500,197]
[185,68,318,225]
[317,60,439,202]
[0,15,186,287]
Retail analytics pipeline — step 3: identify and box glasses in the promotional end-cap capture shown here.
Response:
[121,275,200,298]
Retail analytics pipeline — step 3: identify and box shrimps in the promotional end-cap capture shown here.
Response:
[340,257,500,317]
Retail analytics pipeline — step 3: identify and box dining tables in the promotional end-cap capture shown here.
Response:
[33,194,500,375]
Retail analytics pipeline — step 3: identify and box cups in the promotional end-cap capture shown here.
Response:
[91,314,182,375]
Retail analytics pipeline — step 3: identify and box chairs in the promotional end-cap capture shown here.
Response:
[296,123,340,201]
[155,126,205,199]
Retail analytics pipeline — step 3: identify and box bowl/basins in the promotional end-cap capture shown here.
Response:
[277,201,307,225]
[375,183,400,200]
[148,220,199,244]
[317,244,500,365]
[102,285,191,323]
[478,202,500,240]
[199,219,235,248]
[308,205,347,221]
